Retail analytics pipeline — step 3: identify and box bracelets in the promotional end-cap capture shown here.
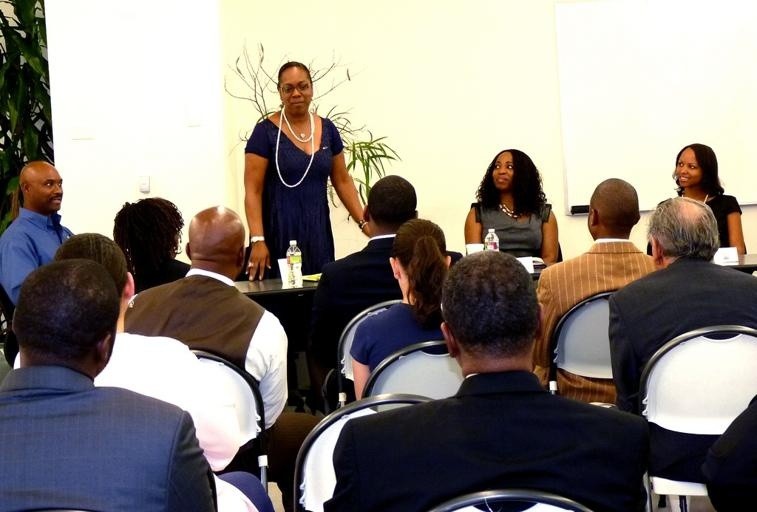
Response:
[248,235,266,243]
[359,221,368,229]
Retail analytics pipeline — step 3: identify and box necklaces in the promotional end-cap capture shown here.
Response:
[272,103,315,188]
[680,193,709,205]
[498,202,531,220]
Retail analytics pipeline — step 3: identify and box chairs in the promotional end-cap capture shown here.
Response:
[291,393,434,511]
[640,325,757,512]
[547,289,619,395]
[191,349,264,435]
[361,341,465,399]
[430,489,594,512]
[338,299,404,406]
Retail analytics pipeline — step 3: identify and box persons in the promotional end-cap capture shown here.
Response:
[343,220,464,399]
[602,196,757,511]
[135,208,325,512]
[467,149,559,282]
[318,176,463,412]
[112,198,190,293]
[0,160,77,369]
[0,258,216,510]
[647,143,748,260]
[13,233,263,472]
[535,178,656,409]
[703,394,757,512]
[244,61,376,416]
[326,249,652,512]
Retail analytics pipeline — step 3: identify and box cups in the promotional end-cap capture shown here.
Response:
[515,256,535,275]
[277,258,289,290]
[467,243,484,256]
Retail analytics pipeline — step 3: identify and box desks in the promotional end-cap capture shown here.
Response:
[709,254,757,275]
[235,258,549,299]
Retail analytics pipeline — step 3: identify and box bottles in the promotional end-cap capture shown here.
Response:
[484,229,501,252]
[286,239,303,288]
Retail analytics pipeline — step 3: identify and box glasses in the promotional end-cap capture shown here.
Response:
[279,81,314,94]
